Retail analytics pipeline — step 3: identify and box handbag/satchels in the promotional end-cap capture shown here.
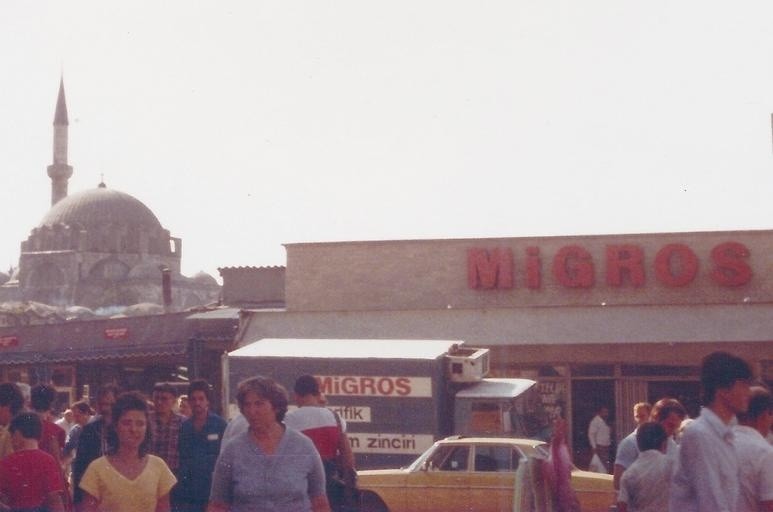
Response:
[324,455,363,512]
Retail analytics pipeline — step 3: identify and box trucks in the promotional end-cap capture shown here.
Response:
[220,338,554,466]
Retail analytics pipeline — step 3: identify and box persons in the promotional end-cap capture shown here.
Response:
[608,381,773,512]
[280,371,356,512]
[175,376,227,512]
[667,349,752,511]
[206,374,331,512]
[586,400,614,474]
[0,371,193,511]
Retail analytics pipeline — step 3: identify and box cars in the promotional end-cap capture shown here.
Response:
[355,435,618,512]
